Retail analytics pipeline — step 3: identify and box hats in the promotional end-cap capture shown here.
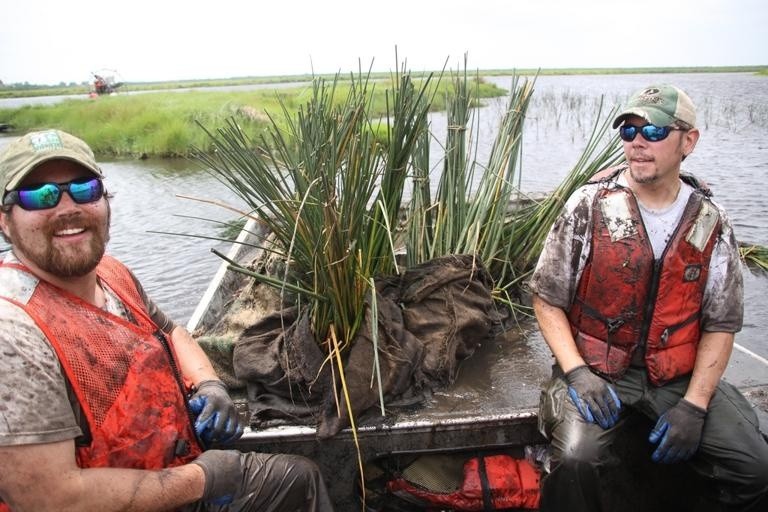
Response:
[613,83,696,128]
[0,129,106,206]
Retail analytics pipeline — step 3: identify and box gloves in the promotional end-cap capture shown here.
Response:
[187,380,243,446]
[565,364,622,430]
[649,398,707,469]
[190,449,243,505]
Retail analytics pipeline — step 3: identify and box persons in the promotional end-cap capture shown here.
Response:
[0,127,339,512]
[526,81,768,511]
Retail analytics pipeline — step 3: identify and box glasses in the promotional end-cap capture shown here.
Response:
[619,125,688,142]
[3,176,103,211]
